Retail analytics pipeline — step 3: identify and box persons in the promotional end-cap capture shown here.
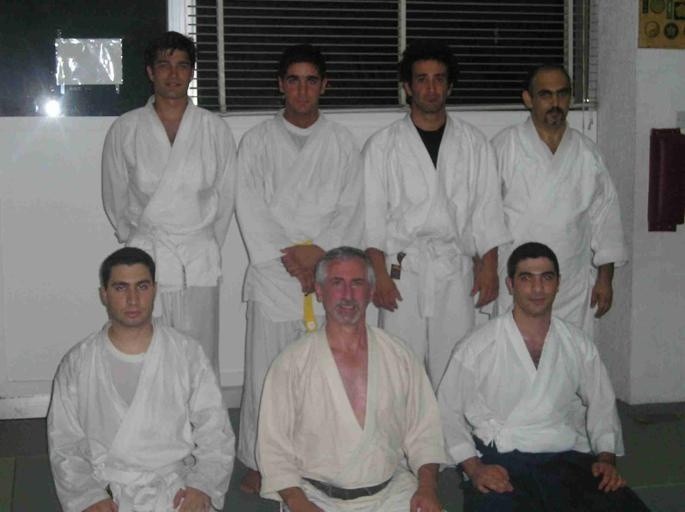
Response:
[235,39,358,499]
[47,246,237,512]
[102,30,237,389]
[253,246,455,511]
[438,241,651,510]
[490,64,630,338]
[359,39,509,401]
